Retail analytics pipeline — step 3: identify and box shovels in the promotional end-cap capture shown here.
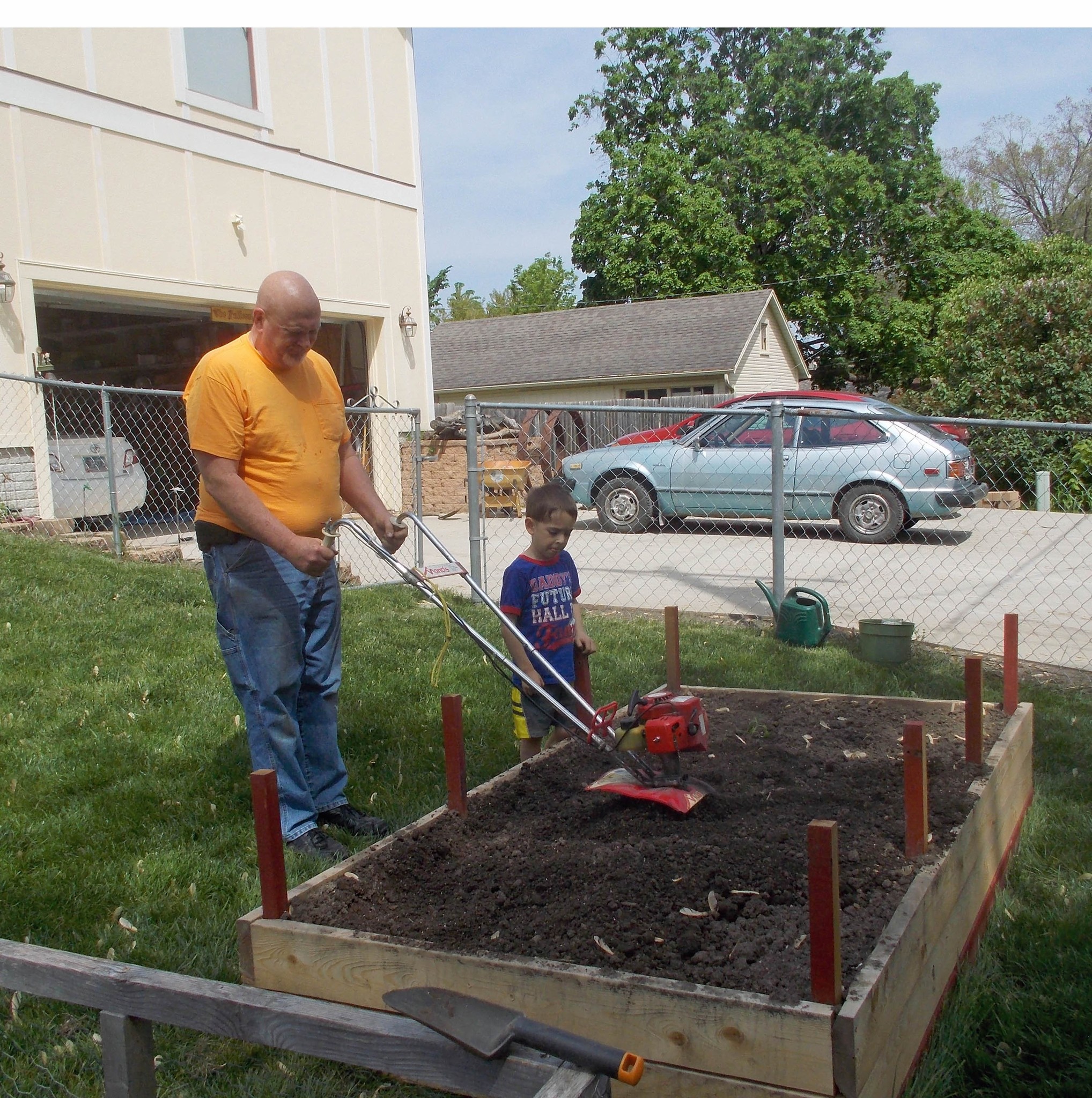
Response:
[380,986,644,1087]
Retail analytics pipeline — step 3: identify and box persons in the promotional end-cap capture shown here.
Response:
[182,270,408,860]
[499,482,597,762]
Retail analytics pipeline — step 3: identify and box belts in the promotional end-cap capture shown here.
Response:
[213,525,244,544]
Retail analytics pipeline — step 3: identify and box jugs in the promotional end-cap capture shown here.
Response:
[754,578,831,647]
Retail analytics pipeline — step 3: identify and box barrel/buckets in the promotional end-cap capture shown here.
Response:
[858,619,915,662]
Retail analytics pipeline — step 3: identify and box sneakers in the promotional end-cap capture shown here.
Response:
[319,804,389,838]
[288,829,351,860]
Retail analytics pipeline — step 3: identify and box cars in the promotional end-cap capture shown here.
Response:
[559,397,990,545]
[604,389,974,449]
